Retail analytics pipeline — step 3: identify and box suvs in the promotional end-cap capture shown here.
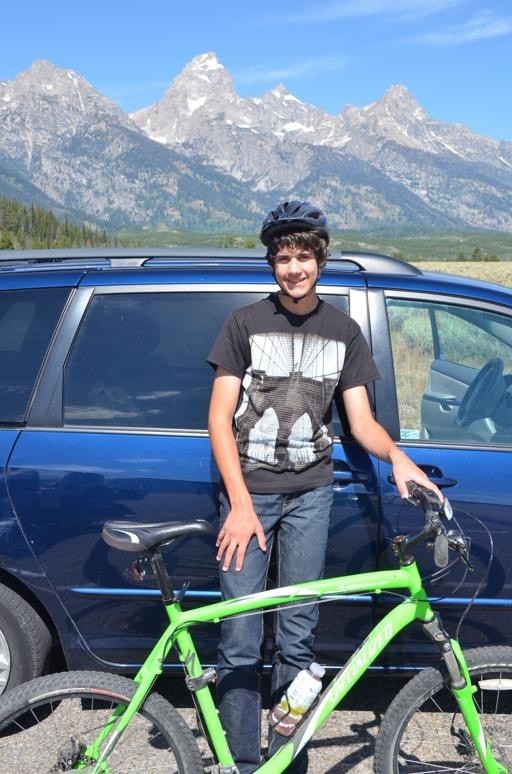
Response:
[0,234,512,736]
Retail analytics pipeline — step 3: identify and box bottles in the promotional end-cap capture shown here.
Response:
[267,662,326,739]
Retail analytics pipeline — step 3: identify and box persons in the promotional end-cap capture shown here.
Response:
[207,200,445,772]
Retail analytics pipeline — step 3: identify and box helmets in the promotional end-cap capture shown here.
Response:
[260,199,328,247]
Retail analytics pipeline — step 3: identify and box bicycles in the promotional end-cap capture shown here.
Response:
[0,461,512,774]
